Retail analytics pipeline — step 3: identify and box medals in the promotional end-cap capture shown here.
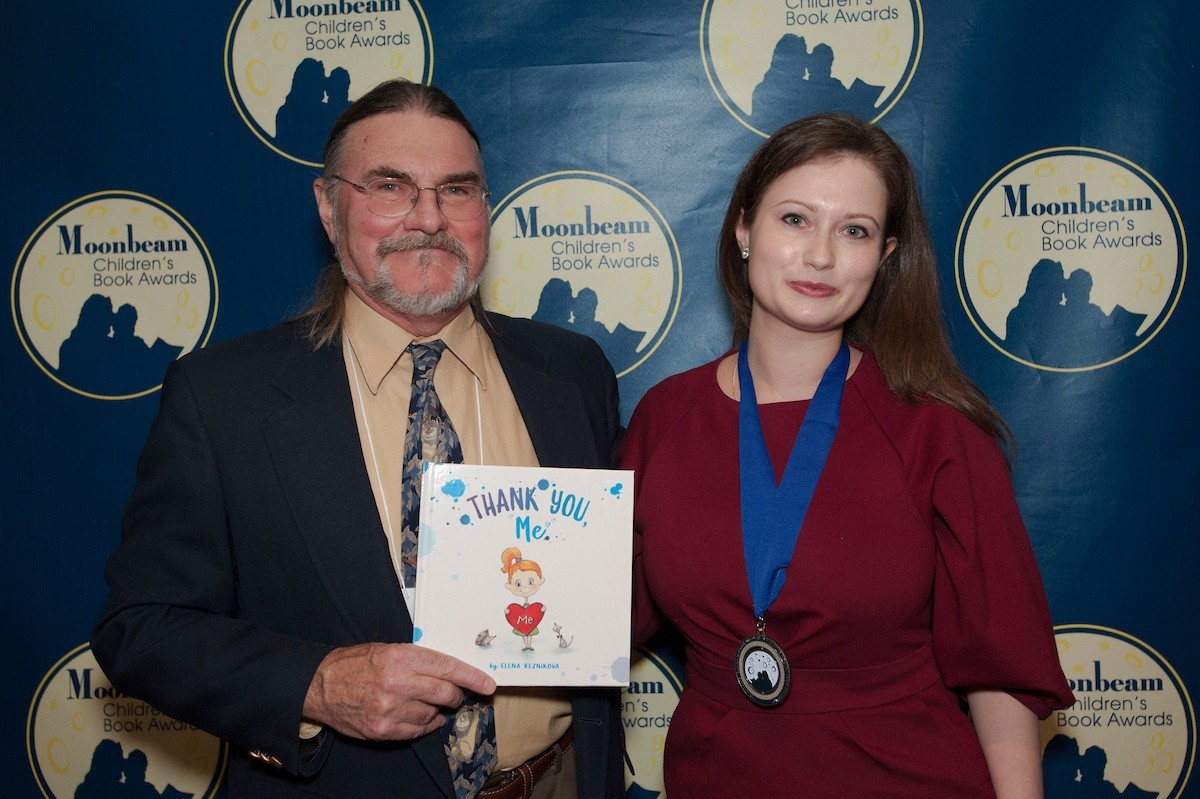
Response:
[735,635,790,708]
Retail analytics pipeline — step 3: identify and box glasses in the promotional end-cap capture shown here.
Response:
[331,174,492,220]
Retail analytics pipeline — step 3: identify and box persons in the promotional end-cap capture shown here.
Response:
[91,80,630,798]
[615,111,1075,799]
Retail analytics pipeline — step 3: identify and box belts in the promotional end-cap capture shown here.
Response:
[470,721,576,799]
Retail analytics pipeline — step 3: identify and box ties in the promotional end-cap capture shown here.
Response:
[401,340,498,798]
